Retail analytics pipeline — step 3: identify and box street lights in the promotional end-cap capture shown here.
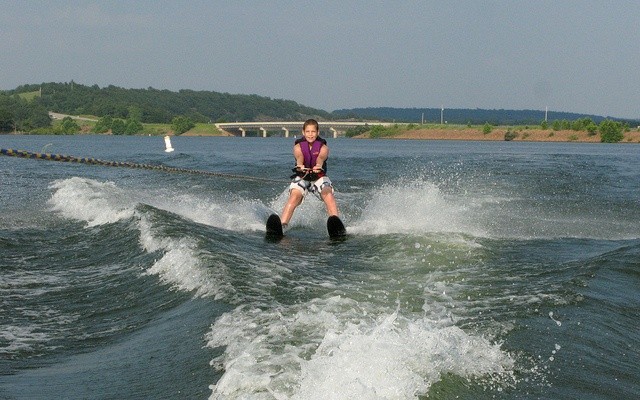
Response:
[441,108,443,124]
[422,112,424,124]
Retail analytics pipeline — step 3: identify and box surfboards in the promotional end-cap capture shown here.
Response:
[266,213,283,238]
[327,215,346,240]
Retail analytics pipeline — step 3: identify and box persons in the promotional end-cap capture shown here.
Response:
[279,119,339,236]
